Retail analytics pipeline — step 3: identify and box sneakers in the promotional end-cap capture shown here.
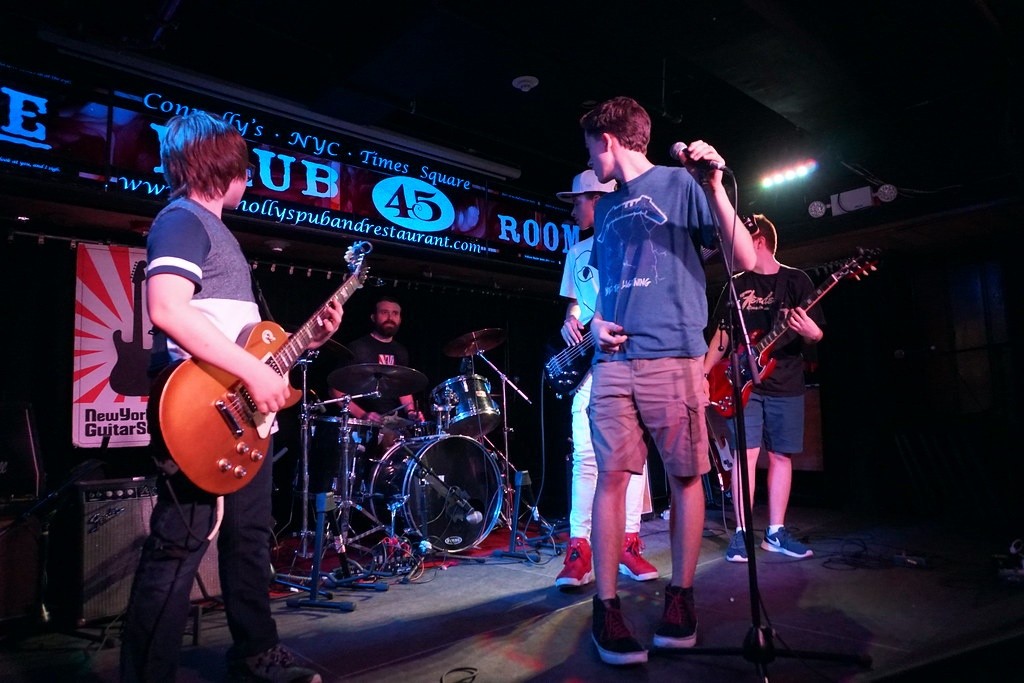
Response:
[727,529,749,563]
[555,537,592,586]
[652,584,698,649]
[591,594,648,665]
[618,533,658,581]
[760,526,813,558]
[228,644,322,683]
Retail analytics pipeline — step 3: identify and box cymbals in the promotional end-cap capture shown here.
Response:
[443,328,507,357]
[326,363,432,399]
[288,322,359,360]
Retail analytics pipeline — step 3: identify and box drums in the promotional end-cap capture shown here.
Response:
[292,413,383,503]
[369,435,504,553]
[426,375,501,437]
[395,421,437,437]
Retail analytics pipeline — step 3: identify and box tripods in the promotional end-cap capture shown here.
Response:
[269,348,486,584]
[653,168,878,683]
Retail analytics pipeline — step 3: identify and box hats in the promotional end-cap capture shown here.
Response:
[556,170,617,203]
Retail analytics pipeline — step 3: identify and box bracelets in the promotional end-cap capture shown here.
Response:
[704,373,709,380]
[813,329,822,342]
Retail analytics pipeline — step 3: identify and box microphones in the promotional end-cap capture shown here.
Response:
[460,357,468,373]
[670,142,734,174]
[466,511,483,525]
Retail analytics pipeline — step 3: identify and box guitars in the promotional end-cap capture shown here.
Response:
[146,240,374,503]
[707,246,881,420]
[541,212,760,401]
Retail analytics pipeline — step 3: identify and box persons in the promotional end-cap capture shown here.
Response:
[332,295,432,557]
[704,213,823,563]
[115,109,344,683]
[579,97,757,665]
[554,169,661,587]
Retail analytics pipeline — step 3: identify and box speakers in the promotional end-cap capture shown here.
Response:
[0,496,39,627]
[69,477,222,624]
[641,472,654,522]
